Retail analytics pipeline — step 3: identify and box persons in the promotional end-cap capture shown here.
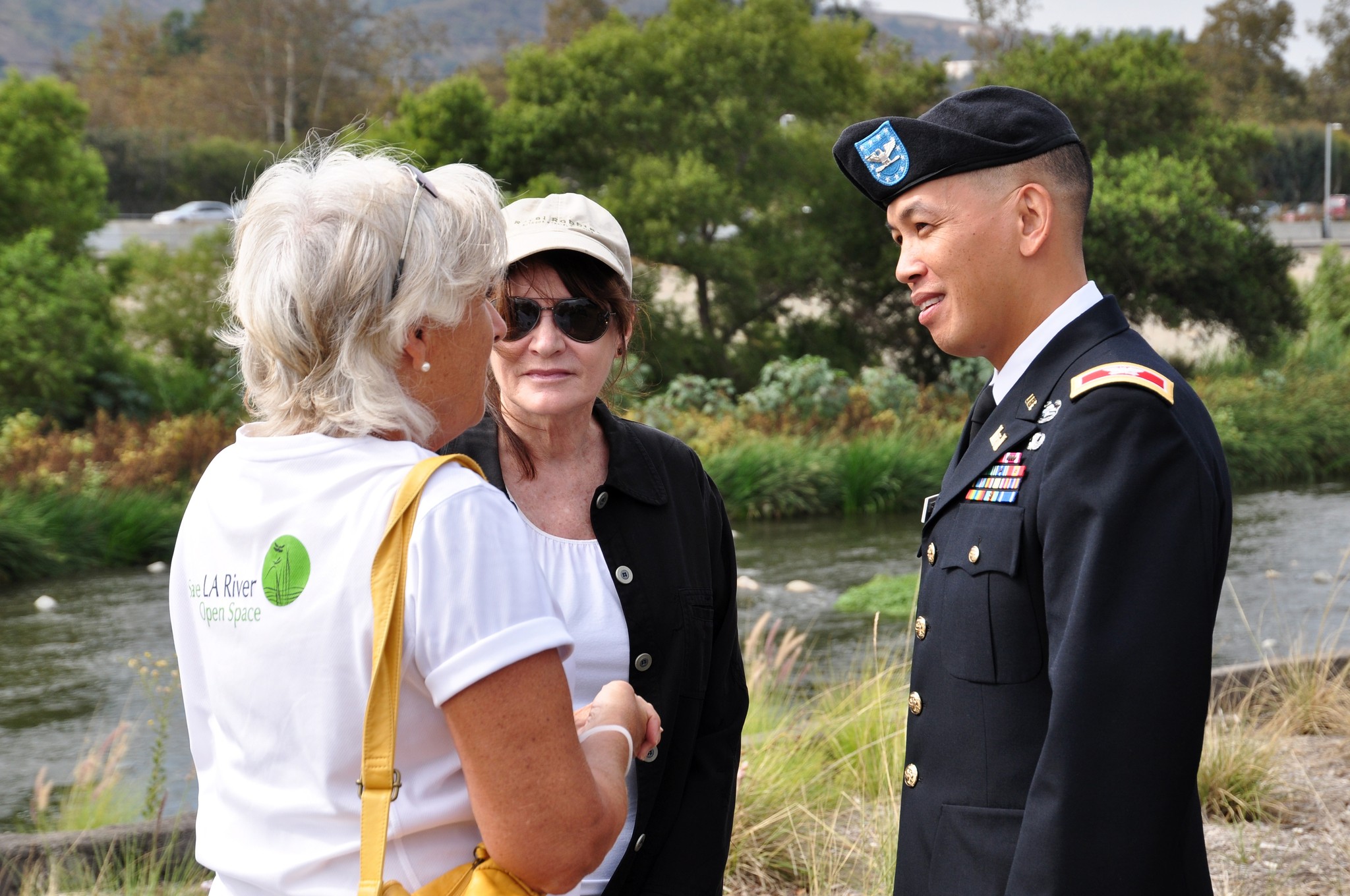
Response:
[434,193,749,896]
[833,88,1232,896]
[169,147,661,896]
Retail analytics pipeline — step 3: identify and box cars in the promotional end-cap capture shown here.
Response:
[150,201,235,227]
[1220,193,1350,222]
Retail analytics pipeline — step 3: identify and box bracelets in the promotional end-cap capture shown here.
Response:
[578,725,633,777]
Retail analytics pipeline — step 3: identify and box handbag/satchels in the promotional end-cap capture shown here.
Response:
[357,453,545,896]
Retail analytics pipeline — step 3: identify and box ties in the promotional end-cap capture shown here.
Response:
[963,385,996,451]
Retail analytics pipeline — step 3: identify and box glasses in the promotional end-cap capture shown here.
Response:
[488,297,617,344]
[391,162,438,298]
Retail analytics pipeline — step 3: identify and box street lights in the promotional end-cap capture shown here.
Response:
[1321,121,1344,242]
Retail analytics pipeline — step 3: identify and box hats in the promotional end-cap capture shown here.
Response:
[493,193,633,298]
[832,85,1081,206]
